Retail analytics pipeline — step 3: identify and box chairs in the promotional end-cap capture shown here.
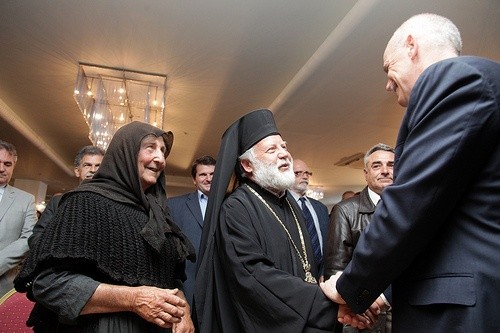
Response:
[0,288,36,333]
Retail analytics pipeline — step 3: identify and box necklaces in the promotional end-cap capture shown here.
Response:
[243,185,317,284]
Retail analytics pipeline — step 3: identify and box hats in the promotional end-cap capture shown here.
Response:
[221,109,281,156]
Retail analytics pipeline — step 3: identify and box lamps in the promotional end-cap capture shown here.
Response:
[73,61,168,151]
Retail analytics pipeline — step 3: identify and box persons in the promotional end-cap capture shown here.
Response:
[320,13,500,333]
[0,109,396,333]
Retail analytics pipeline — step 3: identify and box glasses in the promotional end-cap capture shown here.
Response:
[294,171,313,177]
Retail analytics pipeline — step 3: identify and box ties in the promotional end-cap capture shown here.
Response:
[298,197,322,266]
